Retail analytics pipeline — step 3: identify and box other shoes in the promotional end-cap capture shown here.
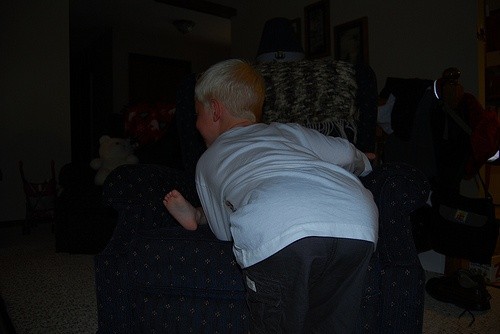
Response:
[428,281,492,327]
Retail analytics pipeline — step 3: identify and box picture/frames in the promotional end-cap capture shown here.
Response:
[334,16,369,73]
[304,0,331,60]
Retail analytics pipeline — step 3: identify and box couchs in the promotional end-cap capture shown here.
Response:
[96,61,430,334]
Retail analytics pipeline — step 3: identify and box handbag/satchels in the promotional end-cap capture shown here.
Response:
[427,158,497,266]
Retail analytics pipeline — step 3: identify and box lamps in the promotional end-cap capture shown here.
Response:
[172,19,193,33]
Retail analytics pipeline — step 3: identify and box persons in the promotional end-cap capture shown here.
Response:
[195,60,379,334]
[335,51,377,88]
[163,190,208,231]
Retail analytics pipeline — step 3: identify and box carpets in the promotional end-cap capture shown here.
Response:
[0,238,500,334]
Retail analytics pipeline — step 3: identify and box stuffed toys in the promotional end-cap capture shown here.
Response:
[90,136,138,185]
